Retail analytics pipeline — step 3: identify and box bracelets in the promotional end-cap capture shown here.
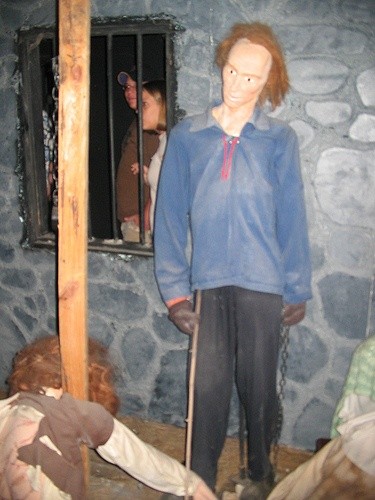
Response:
[164,295,187,309]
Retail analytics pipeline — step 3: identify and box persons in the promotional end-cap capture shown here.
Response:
[41,66,170,251]
[154,25,312,500]
[0,320,375,500]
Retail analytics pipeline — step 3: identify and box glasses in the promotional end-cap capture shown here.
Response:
[122,83,138,90]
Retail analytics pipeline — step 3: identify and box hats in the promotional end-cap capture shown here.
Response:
[117,63,154,85]
